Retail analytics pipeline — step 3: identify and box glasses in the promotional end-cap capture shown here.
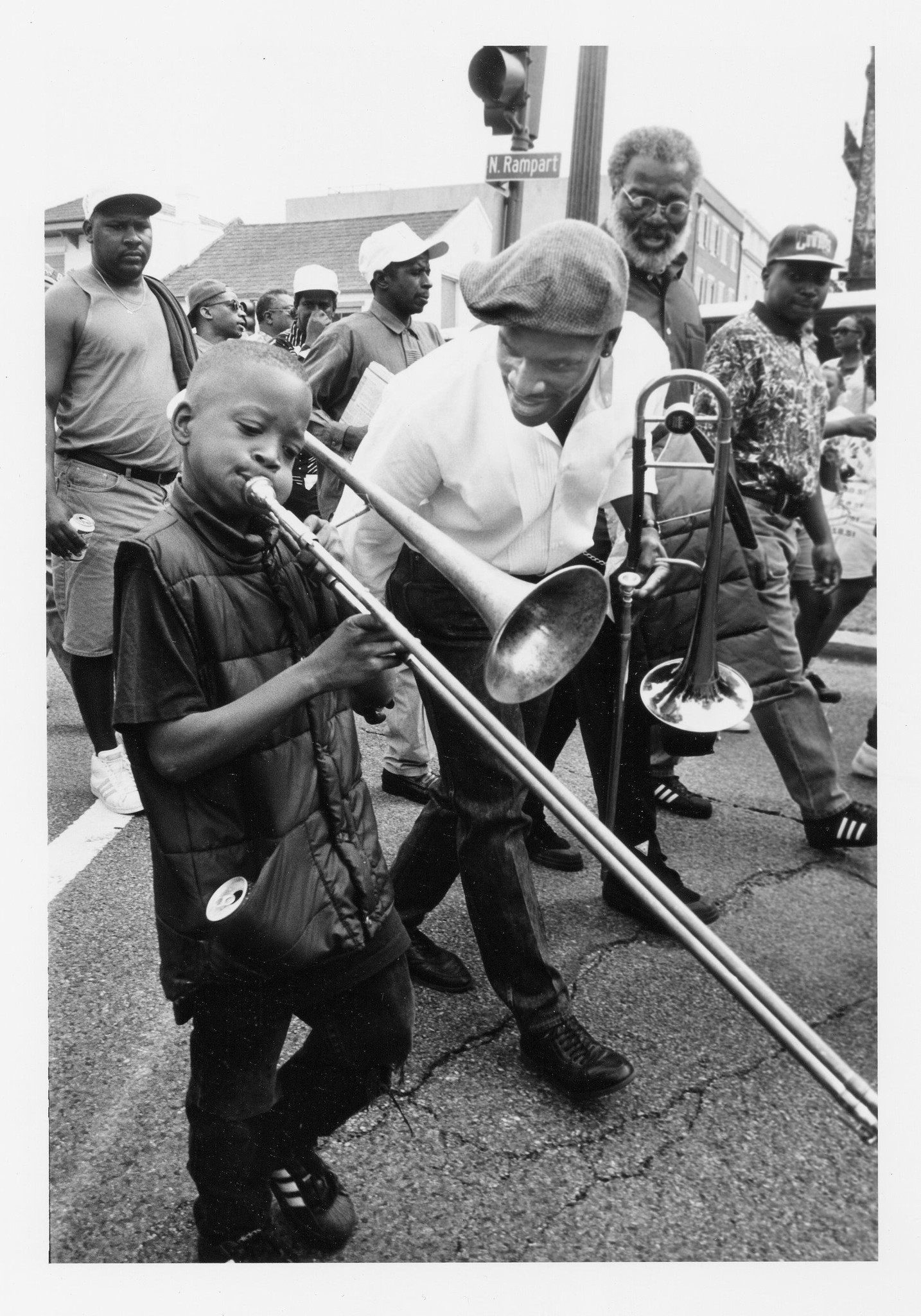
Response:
[620,183,692,224]
[206,300,242,311]
[830,327,859,336]
[269,307,297,319]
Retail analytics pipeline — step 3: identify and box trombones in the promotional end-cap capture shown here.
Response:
[249,472,877,1141]
[598,368,755,886]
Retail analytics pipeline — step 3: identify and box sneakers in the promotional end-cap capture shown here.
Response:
[89,744,145,814]
[523,815,584,871]
[380,768,439,803]
[804,801,877,847]
[269,1145,357,1249]
[601,834,723,924]
[652,777,712,819]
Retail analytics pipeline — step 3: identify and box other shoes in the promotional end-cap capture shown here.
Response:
[806,672,841,701]
[852,740,877,778]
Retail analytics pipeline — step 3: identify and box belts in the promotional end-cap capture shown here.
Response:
[740,488,805,512]
[56,450,178,485]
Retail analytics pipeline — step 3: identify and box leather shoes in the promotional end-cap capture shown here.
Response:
[522,1014,637,1099]
[405,928,474,991]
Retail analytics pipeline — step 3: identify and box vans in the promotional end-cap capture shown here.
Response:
[698,288,876,367]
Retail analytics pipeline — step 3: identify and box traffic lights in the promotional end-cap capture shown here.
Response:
[468,46,548,152]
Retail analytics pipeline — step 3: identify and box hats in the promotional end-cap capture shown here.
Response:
[359,222,449,284]
[186,280,234,327]
[461,216,629,335]
[292,265,339,294]
[81,180,162,220]
[767,223,845,270]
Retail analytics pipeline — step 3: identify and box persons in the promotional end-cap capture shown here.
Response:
[46,127,876,1095]
[106,337,418,1263]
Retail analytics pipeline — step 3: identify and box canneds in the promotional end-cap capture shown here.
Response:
[66,514,95,563]
[205,876,255,921]
